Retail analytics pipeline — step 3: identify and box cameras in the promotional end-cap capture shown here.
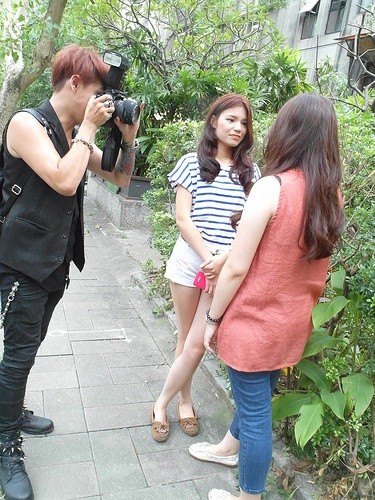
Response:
[94,50,139,130]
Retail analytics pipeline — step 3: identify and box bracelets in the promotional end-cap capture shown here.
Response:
[71,138,93,154]
[206,307,222,322]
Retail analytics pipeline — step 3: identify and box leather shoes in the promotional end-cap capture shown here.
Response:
[19,409,54,434]
[0,455,34,500]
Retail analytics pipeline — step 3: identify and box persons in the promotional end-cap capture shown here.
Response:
[148,92,259,443]
[187,92,348,500]
[0,42,145,500]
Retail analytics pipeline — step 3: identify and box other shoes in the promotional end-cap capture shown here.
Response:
[151,403,171,443]
[188,442,239,468]
[207,487,236,500]
[177,401,200,438]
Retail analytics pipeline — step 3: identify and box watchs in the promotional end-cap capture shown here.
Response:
[204,315,220,325]
[120,137,139,152]
